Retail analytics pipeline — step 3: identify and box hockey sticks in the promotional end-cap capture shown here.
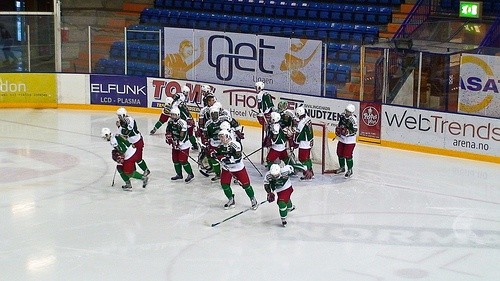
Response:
[243,152,263,177]
[250,109,259,115]
[198,166,209,177]
[112,165,117,186]
[241,126,244,133]
[212,200,268,227]
[172,143,208,169]
[260,108,269,126]
[194,92,201,110]
[212,154,243,186]
[287,147,295,165]
[243,148,263,159]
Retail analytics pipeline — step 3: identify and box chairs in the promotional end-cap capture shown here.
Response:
[94,0,406,98]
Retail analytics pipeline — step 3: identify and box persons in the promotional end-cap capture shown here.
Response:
[116,108,151,177]
[210,129,258,210]
[264,163,294,228]
[165,97,198,151]
[335,104,358,179]
[165,108,195,183]
[150,86,190,136]
[0,23,18,64]
[264,101,315,182]
[195,84,245,184]
[101,127,149,191]
[254,82,274,113]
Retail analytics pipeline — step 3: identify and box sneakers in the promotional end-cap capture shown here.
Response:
[251,196,258,210]
[121,176,149,190]
[334,166,346,176]
[185,173,195,184]
[300,170,312,181]
[149,128,156,136]
[282,218,288,227]
[224,197,235,209]
[344,168,353,179]
[143,168,151,178]
[198,157,222,182]
[172,174,183,182]
[287,205,295,215]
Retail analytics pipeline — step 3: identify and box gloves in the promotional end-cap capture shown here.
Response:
[339,125,348,136]
[195,128,226,161]
[166,134,180,150]
[335,126,342,137]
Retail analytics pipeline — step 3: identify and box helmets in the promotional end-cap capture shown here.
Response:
[99,127,111,142]
[255,81,307,135]
[345,104,357,113]
[270,164,281,179]
[116,108,127,118]
[170,85,233,136]
